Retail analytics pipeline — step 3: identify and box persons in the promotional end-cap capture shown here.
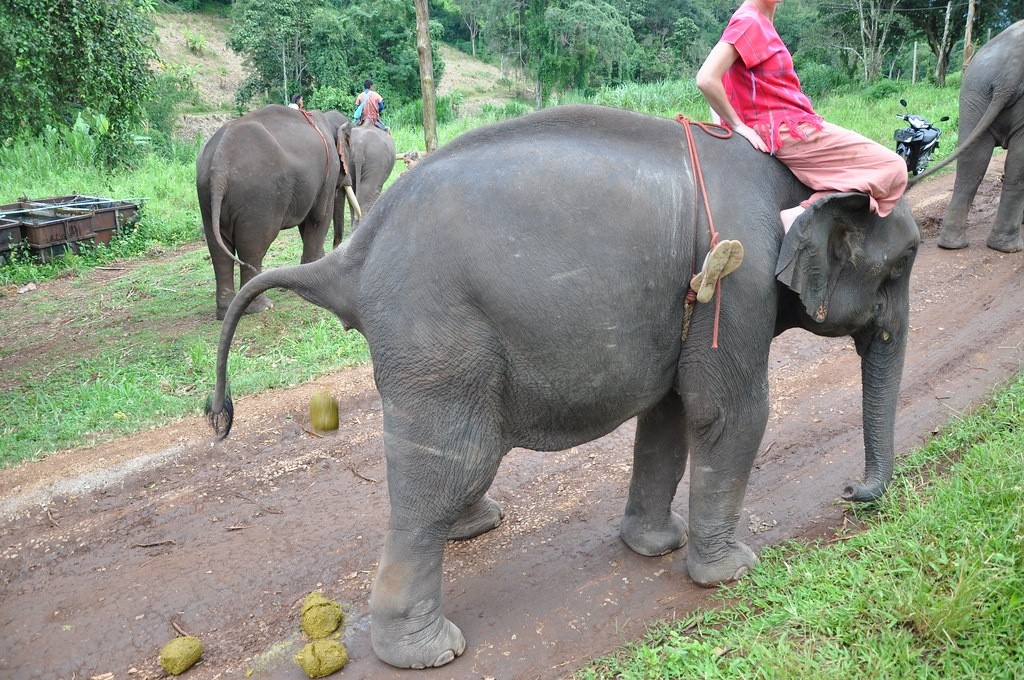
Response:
[356,80,384,128]
[696,0,908,233]
[399,149,422,177]
[288,94,304,111]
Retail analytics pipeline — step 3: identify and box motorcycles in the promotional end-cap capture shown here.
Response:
[894,100,950,177]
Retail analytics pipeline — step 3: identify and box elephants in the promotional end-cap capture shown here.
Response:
[195,103,919,671]
[901,18,1024,253]
